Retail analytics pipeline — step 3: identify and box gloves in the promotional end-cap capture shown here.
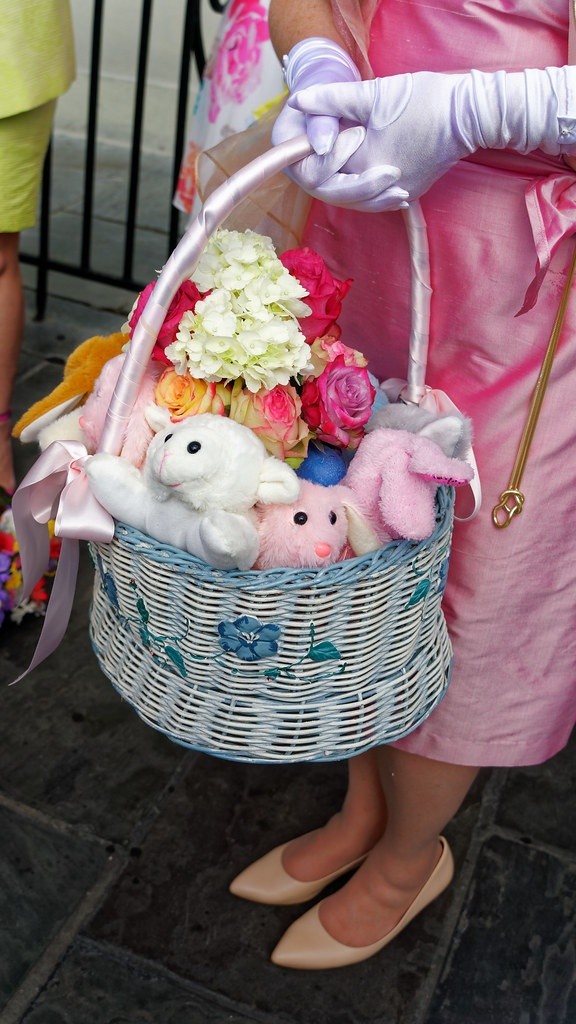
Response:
[268,35,576,213]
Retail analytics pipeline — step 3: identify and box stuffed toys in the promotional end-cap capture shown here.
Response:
[9,327,476,575]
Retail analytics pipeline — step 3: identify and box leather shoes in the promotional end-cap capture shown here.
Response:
[229,840,376,906]
[270,835,455,969]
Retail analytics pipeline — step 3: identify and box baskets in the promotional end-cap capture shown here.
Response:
[85,135,457,767]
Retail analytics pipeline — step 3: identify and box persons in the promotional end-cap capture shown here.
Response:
[0,0,79,519]
[228,0,576,970]
